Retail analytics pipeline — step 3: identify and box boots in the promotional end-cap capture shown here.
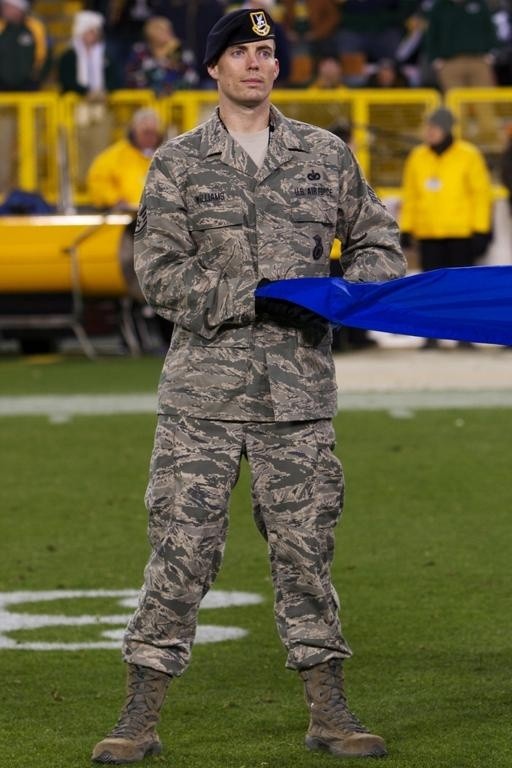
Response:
[91,663,172,763]
[299,660,387,757]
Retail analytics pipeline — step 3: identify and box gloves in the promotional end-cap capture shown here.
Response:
[255,277,326,347]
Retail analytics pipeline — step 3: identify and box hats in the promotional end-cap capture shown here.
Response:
[203,9,277,64]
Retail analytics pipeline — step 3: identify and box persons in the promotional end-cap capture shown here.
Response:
[398,109,496,348]
[330,122,375,353]
[87,107,163,212]
[0,0,512,97]
[84,6,409,767]
